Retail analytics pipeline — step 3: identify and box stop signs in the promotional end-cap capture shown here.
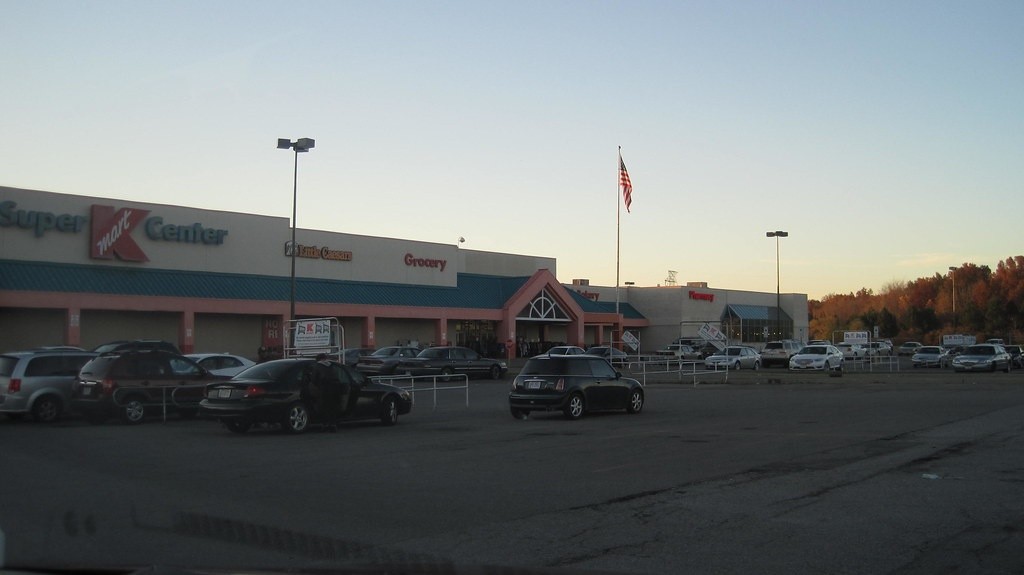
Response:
[506,340,514,350]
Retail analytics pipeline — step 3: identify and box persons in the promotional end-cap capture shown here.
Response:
[518,337,530,358]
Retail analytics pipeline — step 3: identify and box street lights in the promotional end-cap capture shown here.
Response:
[949,267,957,331]
[766,231,789,340]
[277,138,314,357]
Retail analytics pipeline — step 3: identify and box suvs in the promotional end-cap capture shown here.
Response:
[0,339,255,424]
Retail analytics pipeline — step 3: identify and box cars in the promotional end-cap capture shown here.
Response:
[198,359,413,435]
[545,346,629,370]
[761,338,893,366]
[898,338,1024,368]
[952,344,1013,374]
[326,347,420,380]
[656,344,761,371]
[789,345,845,373]
[509,355,644,420]
[397,346,508,382]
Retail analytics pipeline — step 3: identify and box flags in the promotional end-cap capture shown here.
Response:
[620,158,633,213]
